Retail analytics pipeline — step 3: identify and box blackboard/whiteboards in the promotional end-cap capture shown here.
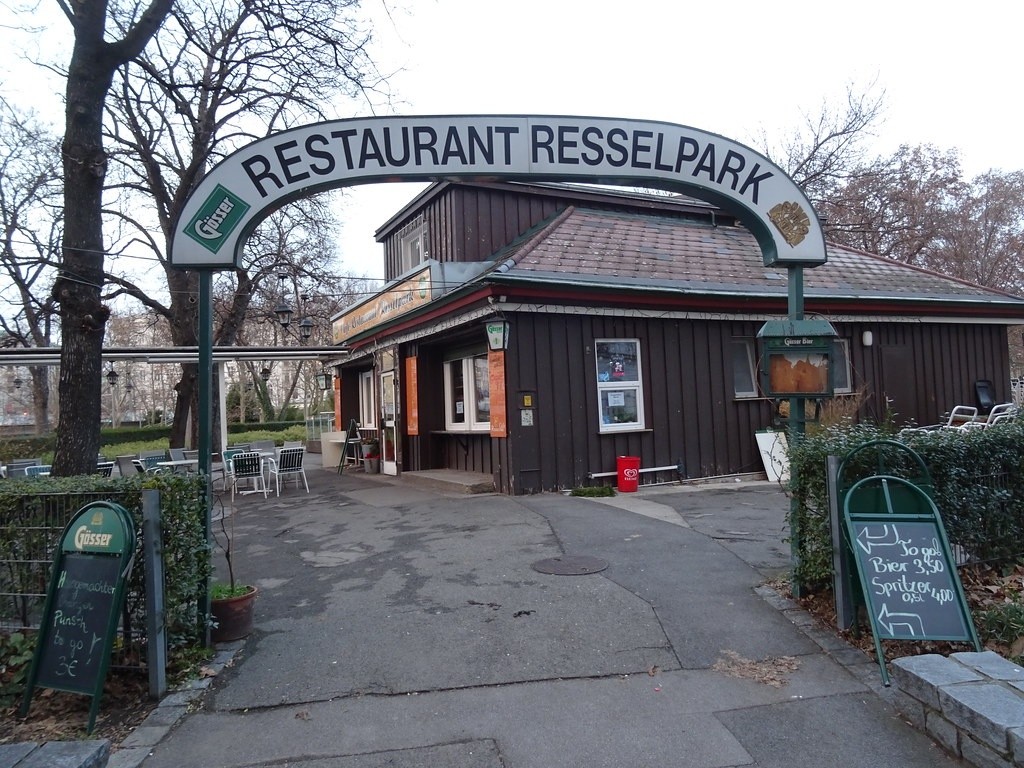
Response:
[28,556,124,696]
[851,521,970,642]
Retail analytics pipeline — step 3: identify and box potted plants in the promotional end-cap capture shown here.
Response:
[203,474,263,641]
[361,436,380,474]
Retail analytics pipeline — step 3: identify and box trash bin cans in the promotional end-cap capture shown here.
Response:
[359,439,380,473]
[616,455,641,492]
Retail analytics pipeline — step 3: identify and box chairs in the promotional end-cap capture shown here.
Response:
[0,440,309,502]
[899,379,1017,438]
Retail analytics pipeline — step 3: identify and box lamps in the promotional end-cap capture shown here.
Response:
[314,365,333,390]
[863,331,873,346]
[481,296,510,352]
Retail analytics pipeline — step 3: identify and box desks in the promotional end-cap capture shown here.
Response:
[239,452,274,496]
[157,459,199,474]
[329,437,379,465]
